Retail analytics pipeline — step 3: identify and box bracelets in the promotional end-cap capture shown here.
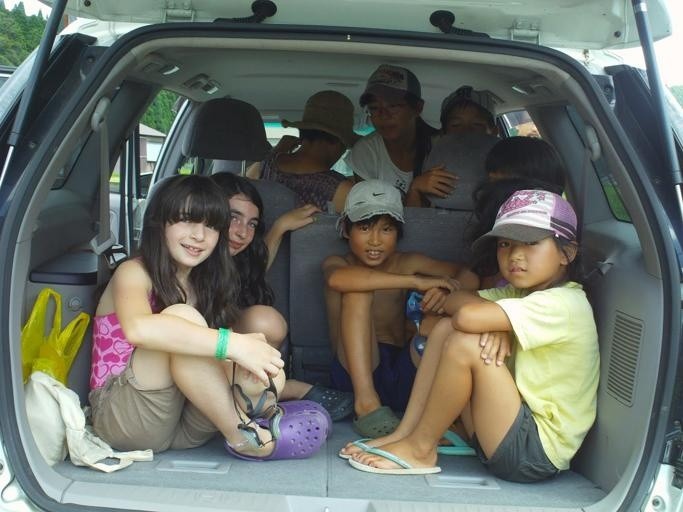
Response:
[215,328,229,360]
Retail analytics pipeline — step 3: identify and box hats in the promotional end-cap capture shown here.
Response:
[280,90,363,149]
[442,84,499,126]
[340,179,405,227]
[465,189,577,252]
[359,63,423,109]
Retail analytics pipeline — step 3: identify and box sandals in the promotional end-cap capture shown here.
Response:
[277,400,334,439]
[354,404,401,441]
[297,382,356,422]
[220,409,329,460]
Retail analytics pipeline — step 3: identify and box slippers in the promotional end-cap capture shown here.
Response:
[436,429,477,456]
[348,446,442,476]
[338,438,374,459]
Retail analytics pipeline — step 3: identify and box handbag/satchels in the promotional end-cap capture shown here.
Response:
[22,370,157,474]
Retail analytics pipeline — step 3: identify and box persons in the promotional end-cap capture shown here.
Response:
[436,178,562,456]
[344,65,437,208]
[211,172,354,421]
[324,186,480,440]
[405,86,500,207]
[245,91,364,214]
[88,175,331,461]
[339,190,600,484]
[487,136,565,191]
